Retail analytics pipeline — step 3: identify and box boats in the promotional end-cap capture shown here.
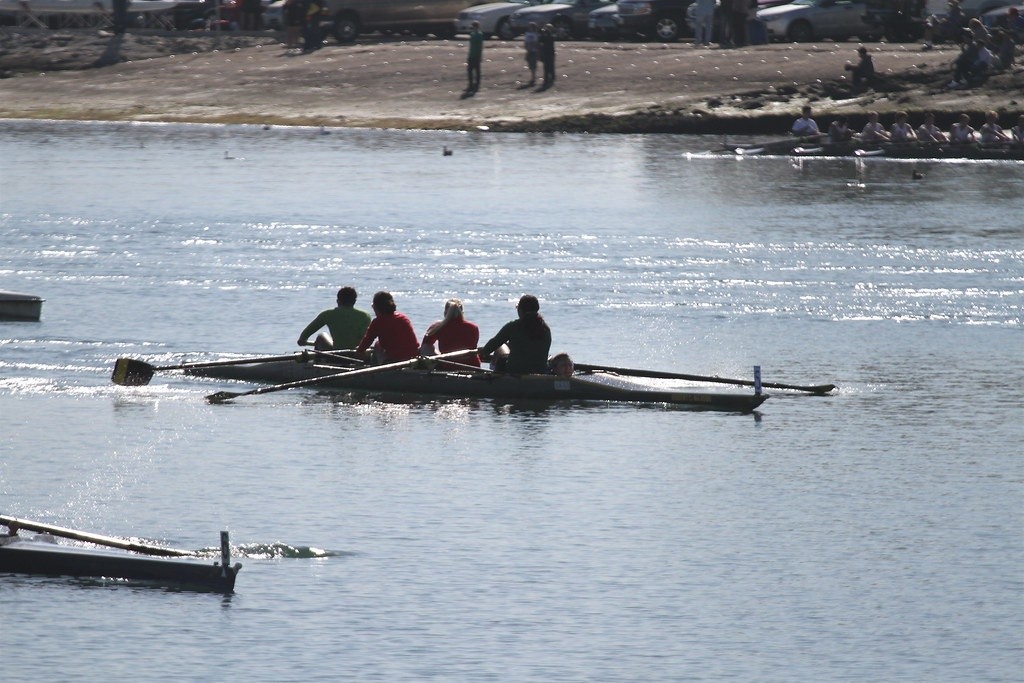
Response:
[0,534,242,588]
[185,357,770,412]
[0,292,46,321]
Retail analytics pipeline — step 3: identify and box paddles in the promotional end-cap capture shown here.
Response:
[0,515,185,556]
[303,341,315,346]
[480,354,836,392]
[732,129,1017,155]
[111,348,372,386]
[205,347,478,400]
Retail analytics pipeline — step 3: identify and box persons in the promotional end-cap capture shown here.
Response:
[298,288,372,350]
[828,113,854,143]
[478,296,552,373]
[792,106,819,143]
[355,292,419,366]
[280,0,326,49]
[920,0,1024,91]
[421,301,481,371]
[523,22,556,91]
[692,0,760,48]
[862,110,1024,145]
[466,20,483,92]
[844,47,874,87]
[240,0,258,30]
[112,0,129,36]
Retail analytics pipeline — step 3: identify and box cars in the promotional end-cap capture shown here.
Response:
[979,2,1024,29]
[587,4,627,40]
[510,0,615,40]
[453,0,551,42]
[173,0,484,44]
[617,0,721,42]
[754,0,884,42]
